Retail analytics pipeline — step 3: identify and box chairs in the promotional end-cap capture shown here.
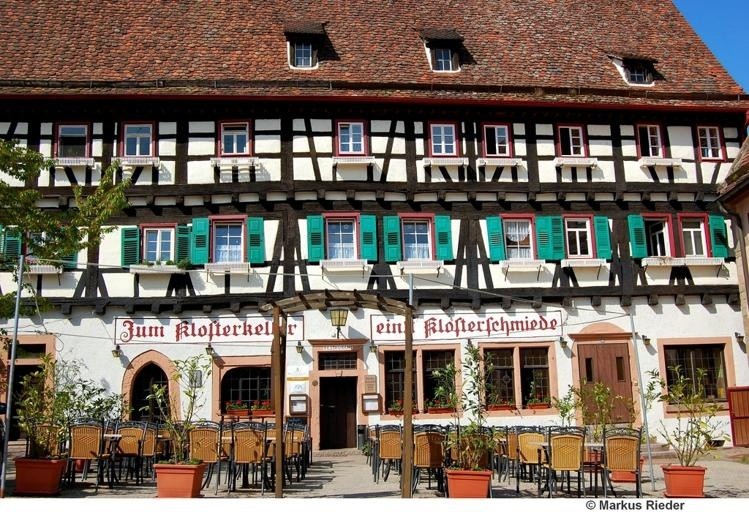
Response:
[364,424,643,498]
[34,418,312,497]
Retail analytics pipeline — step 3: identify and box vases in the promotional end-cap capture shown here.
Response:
[388,407,418,415]
[252,409,273,416]
[226,408,248,417]
[428,406,456,414]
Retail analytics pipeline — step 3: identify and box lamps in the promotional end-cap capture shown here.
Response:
[369,340,377,353]
[330,308,348,338]
[296,341,303,353]
[112,345,120,357]
[559,337,567,348]
[642,334,650,345]
[735,332,744,342]
[206,342,213,355]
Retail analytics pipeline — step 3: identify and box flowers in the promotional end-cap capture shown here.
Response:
[394,400,415,409]
[251,400,270,410]
[226,400,247,409]
[429,400,454,409]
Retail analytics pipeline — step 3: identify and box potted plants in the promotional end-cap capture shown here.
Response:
[655,366,730,498]
[12,353,104,493]
[487,398,515,410]
[525,382,550,409]
